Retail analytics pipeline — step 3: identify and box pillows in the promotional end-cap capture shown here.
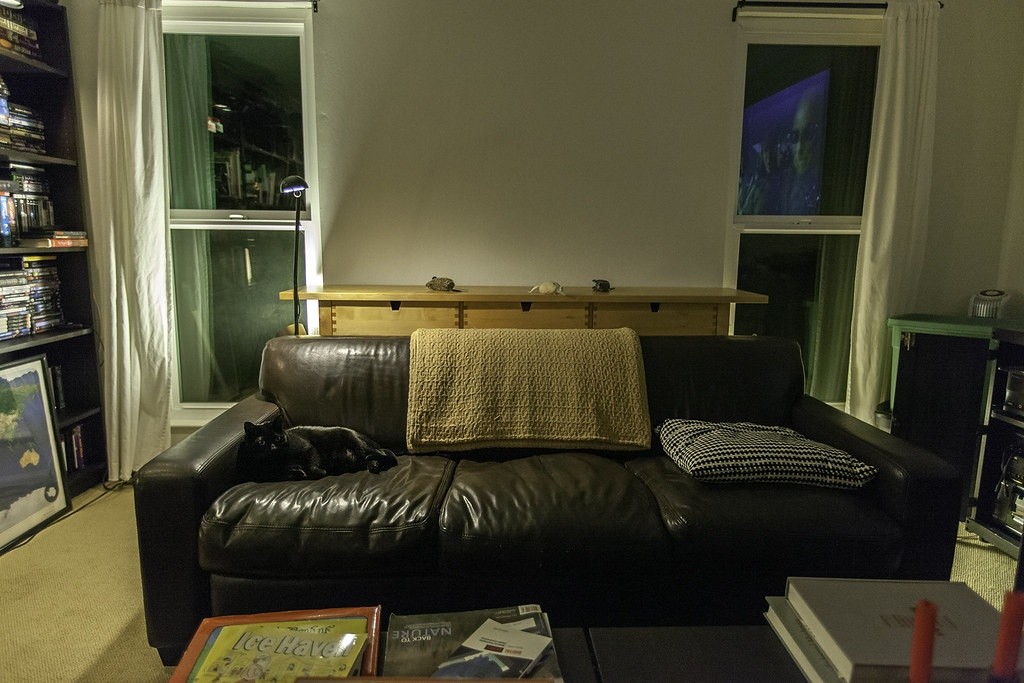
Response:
[653,416,872,495]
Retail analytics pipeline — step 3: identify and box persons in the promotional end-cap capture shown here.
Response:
[741,87,820,215]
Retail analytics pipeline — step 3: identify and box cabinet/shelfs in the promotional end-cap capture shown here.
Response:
[964,327,1024,559]
[886,312,1024,413]
[0,1,111,500]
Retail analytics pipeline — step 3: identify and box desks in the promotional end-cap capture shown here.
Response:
[279,286,770,337]
[167,625,812,683]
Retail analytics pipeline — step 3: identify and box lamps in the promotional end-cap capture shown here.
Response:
[280,175,309,337]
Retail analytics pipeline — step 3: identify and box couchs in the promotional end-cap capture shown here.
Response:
[133,333,965,672]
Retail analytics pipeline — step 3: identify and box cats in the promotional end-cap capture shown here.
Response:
[244,415,398,479]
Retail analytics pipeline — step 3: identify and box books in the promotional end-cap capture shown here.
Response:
[0,256,65,341]
[761,575,1024,683]
[166,602,571,683]
[0,163,88,248]
[0,78,46,154]
[50,361,88,475]
[0,5,42,60]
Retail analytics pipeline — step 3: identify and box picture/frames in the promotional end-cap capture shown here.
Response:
[0,353,74,547]
